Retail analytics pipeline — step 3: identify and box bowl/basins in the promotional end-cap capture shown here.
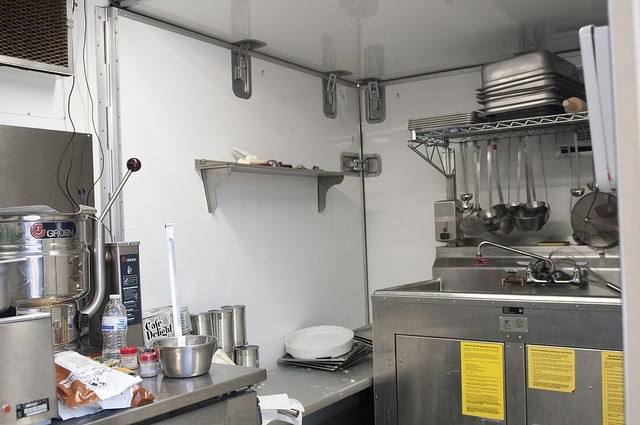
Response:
[153,337,216,377]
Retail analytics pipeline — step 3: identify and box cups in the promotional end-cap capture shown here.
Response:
[235,346,261,393]
[190,305,247,365]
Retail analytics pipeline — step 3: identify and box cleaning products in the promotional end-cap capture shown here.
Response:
[562,97,586,113]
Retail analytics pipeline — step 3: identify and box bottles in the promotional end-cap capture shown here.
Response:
[119,344,139,371]
[137,352,159,378]
[101,294,129,361]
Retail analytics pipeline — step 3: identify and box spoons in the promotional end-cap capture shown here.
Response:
[587,157,599,191]
[571,133,585,197]
[457,141,512,236]
